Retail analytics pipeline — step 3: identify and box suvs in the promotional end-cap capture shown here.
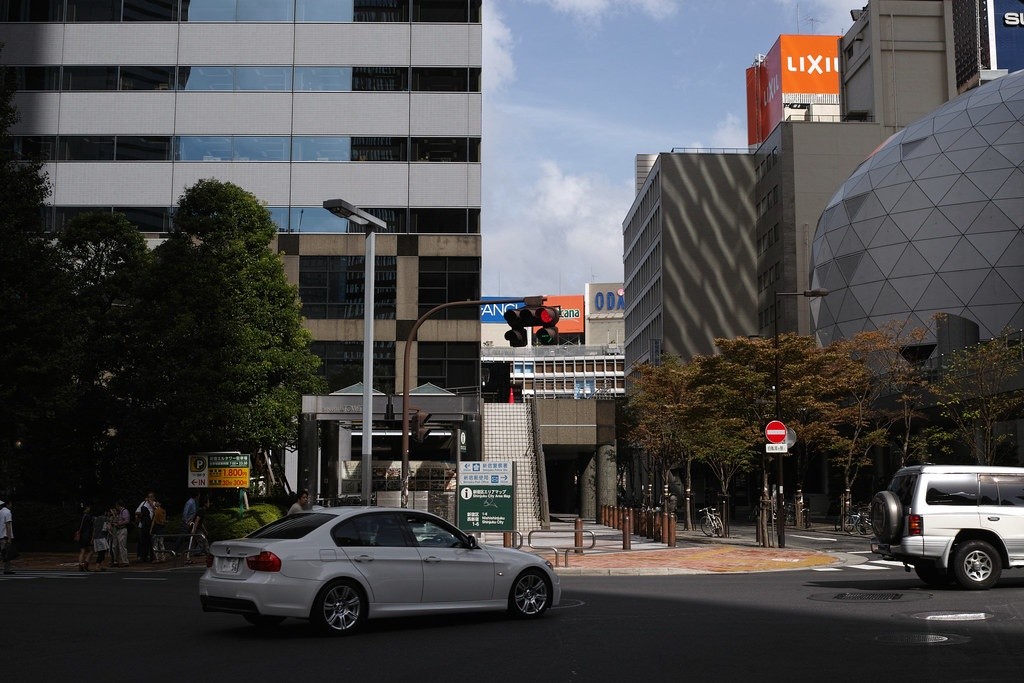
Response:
[869,463,1024,591]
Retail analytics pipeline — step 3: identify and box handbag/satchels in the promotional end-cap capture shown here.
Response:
[3,544,19,562]
[74,531,80,542]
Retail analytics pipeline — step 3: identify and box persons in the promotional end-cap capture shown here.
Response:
[0,497,16,575]
[78,499,131,572]
[134,492,167,563]
[287,490,308,514]
[171,491,210,557]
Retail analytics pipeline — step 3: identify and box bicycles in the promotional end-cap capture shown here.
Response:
[698,504,725,538]
[835,502,874,536]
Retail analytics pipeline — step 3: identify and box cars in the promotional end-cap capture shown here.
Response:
[197,505,563,638]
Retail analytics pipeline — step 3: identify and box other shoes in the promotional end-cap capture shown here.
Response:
[120,563,129,567]
[3,571,16,574]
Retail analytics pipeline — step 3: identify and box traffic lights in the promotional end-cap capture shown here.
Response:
[536,326,558,345]
[503,307,559,328]
[503,328,527,347]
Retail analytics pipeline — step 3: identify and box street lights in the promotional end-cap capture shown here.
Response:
[773,288,831,551]
[322,198,389,507]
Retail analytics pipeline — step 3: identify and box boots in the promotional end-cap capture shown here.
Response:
[80,560,89,572]
[95,563,104,572]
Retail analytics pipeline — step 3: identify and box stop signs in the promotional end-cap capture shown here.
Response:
[765,420,788,444]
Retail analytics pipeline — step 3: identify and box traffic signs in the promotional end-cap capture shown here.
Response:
[187,451,251,488]
